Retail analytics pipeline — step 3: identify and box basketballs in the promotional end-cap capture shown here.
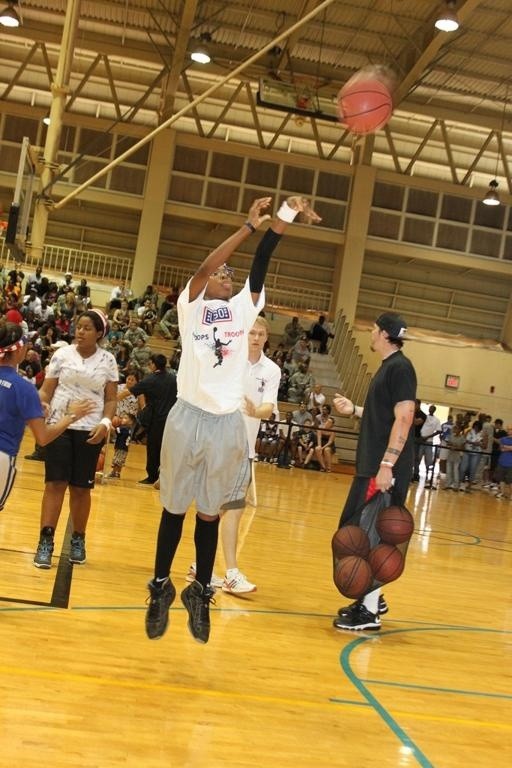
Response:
[368,543,404,582]
[333,557,373,597]
[333,525,369,561]
[376,506,413,543]
[96,450,105,472]
[340,78,391,135]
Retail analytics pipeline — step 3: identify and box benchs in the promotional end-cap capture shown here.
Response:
[80,286,361,465]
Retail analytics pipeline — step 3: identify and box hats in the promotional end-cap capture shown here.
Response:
[376,311,418,340]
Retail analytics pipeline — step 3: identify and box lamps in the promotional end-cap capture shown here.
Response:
[1,0,21,28]
[435,0,460,32]
[190,32,213,64]
[42,106,50,125]
[482,82,512,206]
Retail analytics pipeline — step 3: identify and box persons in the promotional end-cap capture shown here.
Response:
[181,316,283,598]
[410,399,511,507]
[328,310,423,632]
[0,264,336,489]
[143,196,322,646]
[32,310,120,571]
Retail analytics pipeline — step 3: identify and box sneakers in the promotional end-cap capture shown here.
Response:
[181,580,216,644]
[334,604,382,630]
[185,563,224,588]
[144,577,176,639]
[338,594,388,618]
[221,572,257,593]
[68,538,86,564]
[33,538,54,569]
